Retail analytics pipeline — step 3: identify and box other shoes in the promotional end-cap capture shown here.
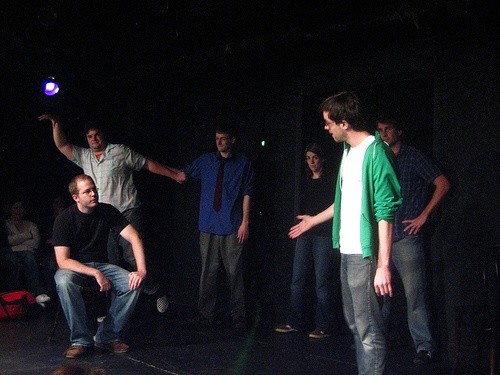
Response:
[231,320,248,336]
[156,287,169,313]
[34,293,51,304]
[273,324,303,333]
[96,316,106,323]
[186,312,216,324]
[309,330,333,338]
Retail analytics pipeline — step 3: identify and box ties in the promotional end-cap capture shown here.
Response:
[212,156,226,211]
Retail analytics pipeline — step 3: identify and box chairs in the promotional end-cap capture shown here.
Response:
[451,240,500,375]
[47,238,109,342]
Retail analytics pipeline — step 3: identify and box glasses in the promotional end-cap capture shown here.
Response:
[324,121,334,127]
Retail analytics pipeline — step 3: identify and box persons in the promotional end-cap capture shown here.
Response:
[272,143,337,339]
[0,194,71,293]
[143,120,254,329]
[37,114,190,293]
[350,113,452,366]
[52,175,148,359]
[287,92,405,375]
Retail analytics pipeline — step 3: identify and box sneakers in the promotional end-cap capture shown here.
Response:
[65,344,95,359]
[95,340,129,353]
[412,349,440,364]
